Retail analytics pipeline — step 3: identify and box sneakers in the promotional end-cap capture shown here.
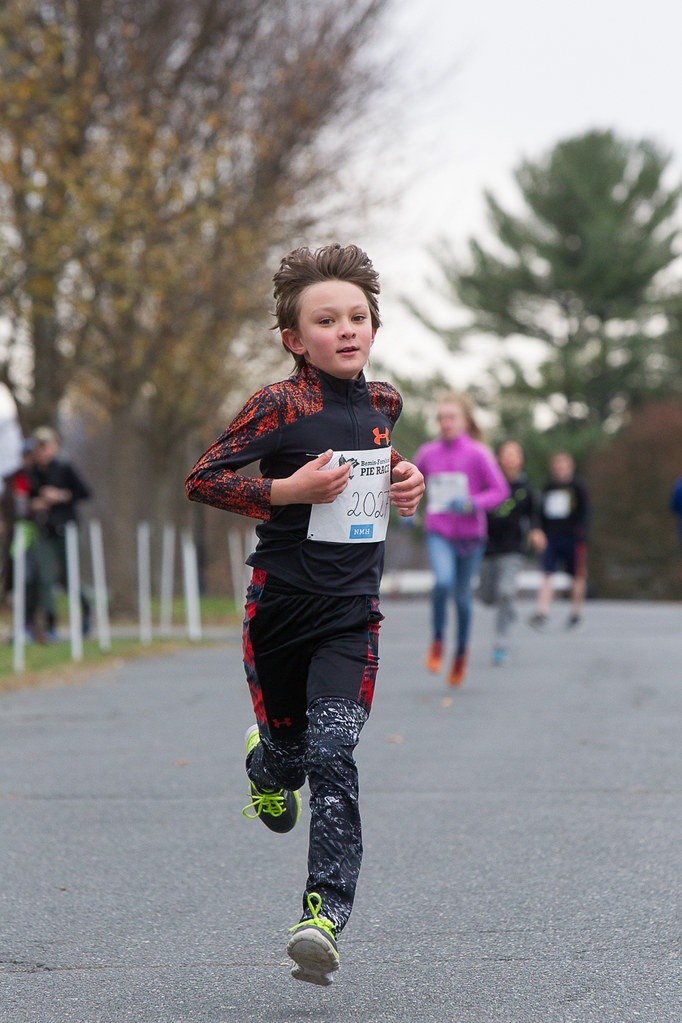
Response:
[245,723,302,833]
[426,639,444,674]
[285,916,341,986]
[447,657,465,687]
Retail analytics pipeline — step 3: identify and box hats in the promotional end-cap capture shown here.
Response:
[31,424,57,443]
[21,436,37,454]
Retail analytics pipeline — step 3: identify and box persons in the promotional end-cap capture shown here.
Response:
[0,424,94,646]
[527,449,584,631]
[411,394,511,689]
[476,435,548,669]
[186,243,426,987]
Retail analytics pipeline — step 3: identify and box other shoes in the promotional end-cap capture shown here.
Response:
[492,646,507,665]
[82,607,93,638]
[531,615,547,627]
[21,624,59,647]
[567,614,581,627]
